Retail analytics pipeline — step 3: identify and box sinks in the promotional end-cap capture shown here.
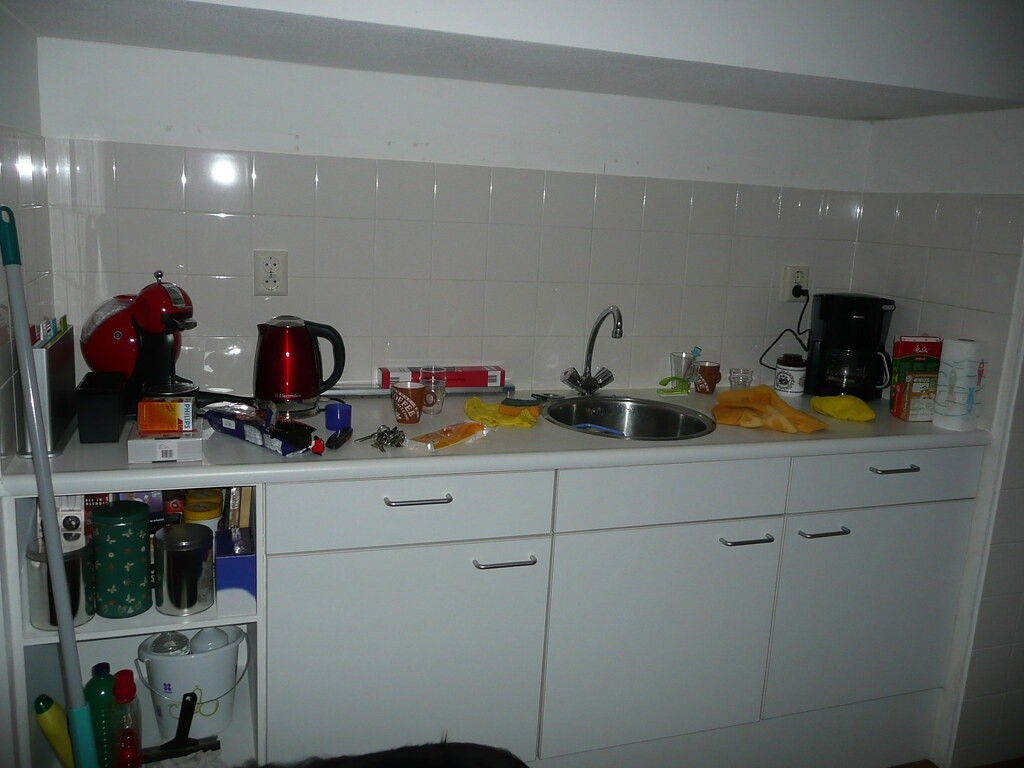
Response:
[541,395,716,441]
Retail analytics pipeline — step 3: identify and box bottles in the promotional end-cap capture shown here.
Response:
[191,627,229,653]
[33,694,75,768]
[84,663,143,768]
[773,357,805,398]
[148,630,190,655]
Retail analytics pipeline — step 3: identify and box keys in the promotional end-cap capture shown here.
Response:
[354,424,406,454]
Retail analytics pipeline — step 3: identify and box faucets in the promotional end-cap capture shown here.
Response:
[560,305,623,397]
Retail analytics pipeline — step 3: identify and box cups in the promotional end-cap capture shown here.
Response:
[728,368,754,391]
[670,351,700,388]
[90,499,154,620]
[25,535,95,631]
[693,359,722,394]
[420,365,447,415]
[389,382,436,424]
[152,522,215,617]
[324,404,352,430]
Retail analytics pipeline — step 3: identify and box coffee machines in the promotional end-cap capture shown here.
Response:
[808,291,896,401]
[80,268,200,413]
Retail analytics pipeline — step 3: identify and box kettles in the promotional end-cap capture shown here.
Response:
[252,314,346,421]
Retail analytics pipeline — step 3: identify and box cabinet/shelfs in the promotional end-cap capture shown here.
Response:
[538,455,791,768]
[2,484,263,768]
[760,444,986,768]
[264,470,557,768]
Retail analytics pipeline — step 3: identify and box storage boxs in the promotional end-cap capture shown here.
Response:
[378,367,505,389]
[889,335,943,421]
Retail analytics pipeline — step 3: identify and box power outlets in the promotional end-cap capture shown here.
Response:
[253,249,288,296]
[781,265,810,302]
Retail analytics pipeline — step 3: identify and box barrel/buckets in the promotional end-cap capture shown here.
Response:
[134,626,253,741]
